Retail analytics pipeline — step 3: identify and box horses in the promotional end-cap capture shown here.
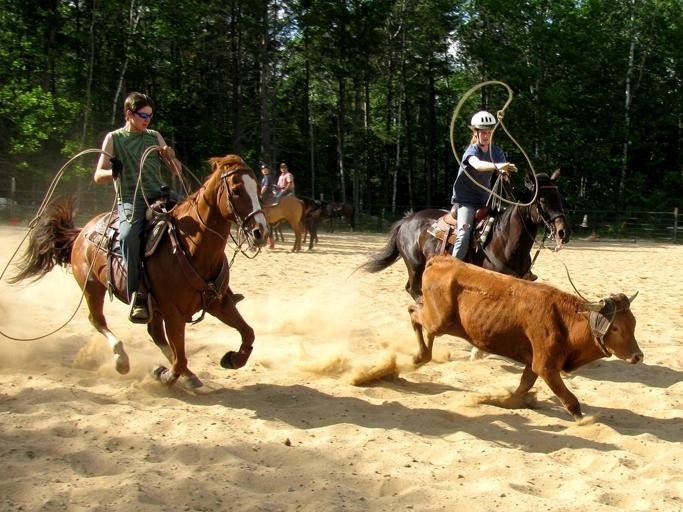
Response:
[345,167,572,362]
[6,153,272,389]
[256,182,356,253]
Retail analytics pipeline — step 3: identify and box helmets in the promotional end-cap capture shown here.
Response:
[471,110,496,130]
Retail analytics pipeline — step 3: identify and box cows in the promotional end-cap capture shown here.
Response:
[407,253,644,422]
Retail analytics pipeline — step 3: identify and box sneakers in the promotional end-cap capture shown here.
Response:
[131,303,148,319]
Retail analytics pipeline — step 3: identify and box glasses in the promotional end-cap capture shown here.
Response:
[128,107,154,118]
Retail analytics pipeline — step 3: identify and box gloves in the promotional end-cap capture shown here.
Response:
[497,162,519,174]
[110,157,122,179]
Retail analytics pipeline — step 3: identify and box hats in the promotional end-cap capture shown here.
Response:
[261,164,269,168]
[280,163,288,168]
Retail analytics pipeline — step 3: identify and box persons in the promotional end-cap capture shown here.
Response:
[88,91,184,323]
[449,108,519,266]
[256,162,272,201]
[267,163,296,202]
